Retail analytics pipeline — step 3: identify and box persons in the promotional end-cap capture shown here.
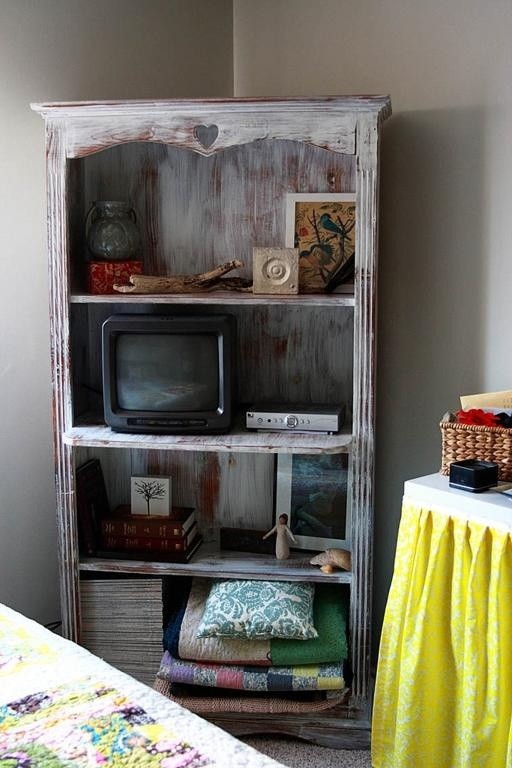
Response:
[263,512,298,559]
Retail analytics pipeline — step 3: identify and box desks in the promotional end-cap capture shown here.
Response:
[362,466,511,767]
[0,599,297,767]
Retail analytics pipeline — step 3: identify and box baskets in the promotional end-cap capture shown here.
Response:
[440,419,511,486]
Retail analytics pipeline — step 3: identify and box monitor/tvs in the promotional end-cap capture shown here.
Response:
[99,314,233,434]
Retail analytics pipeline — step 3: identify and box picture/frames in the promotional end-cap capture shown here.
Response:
[285,193,356,294]
[270,448,353,557]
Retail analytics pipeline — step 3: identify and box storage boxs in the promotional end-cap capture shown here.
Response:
[85,258,144,295]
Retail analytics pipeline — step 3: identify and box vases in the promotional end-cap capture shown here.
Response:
[86,198,141,261]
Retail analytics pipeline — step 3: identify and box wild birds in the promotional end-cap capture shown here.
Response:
[317,213,352,242]
[299,244,336,264]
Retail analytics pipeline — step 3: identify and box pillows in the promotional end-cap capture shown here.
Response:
[193,579,321,640]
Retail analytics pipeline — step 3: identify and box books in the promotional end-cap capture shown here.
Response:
[100,521,198,553]
[96,533,205,563]
[101,505,195,539]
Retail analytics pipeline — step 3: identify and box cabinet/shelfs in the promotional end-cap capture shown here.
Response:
[27,95,398,751]
